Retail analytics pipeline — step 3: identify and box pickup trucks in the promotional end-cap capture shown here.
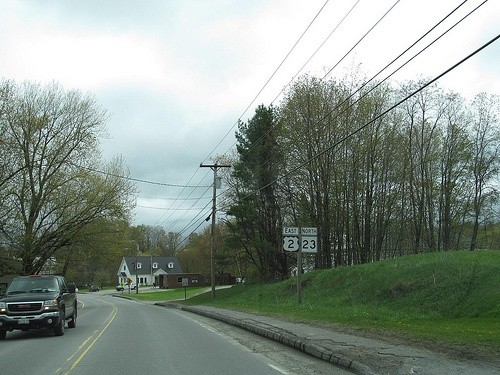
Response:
[116,283,137,291]
[0,274,78,339]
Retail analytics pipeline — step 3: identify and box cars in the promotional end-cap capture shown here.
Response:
[89,285,100,292]
